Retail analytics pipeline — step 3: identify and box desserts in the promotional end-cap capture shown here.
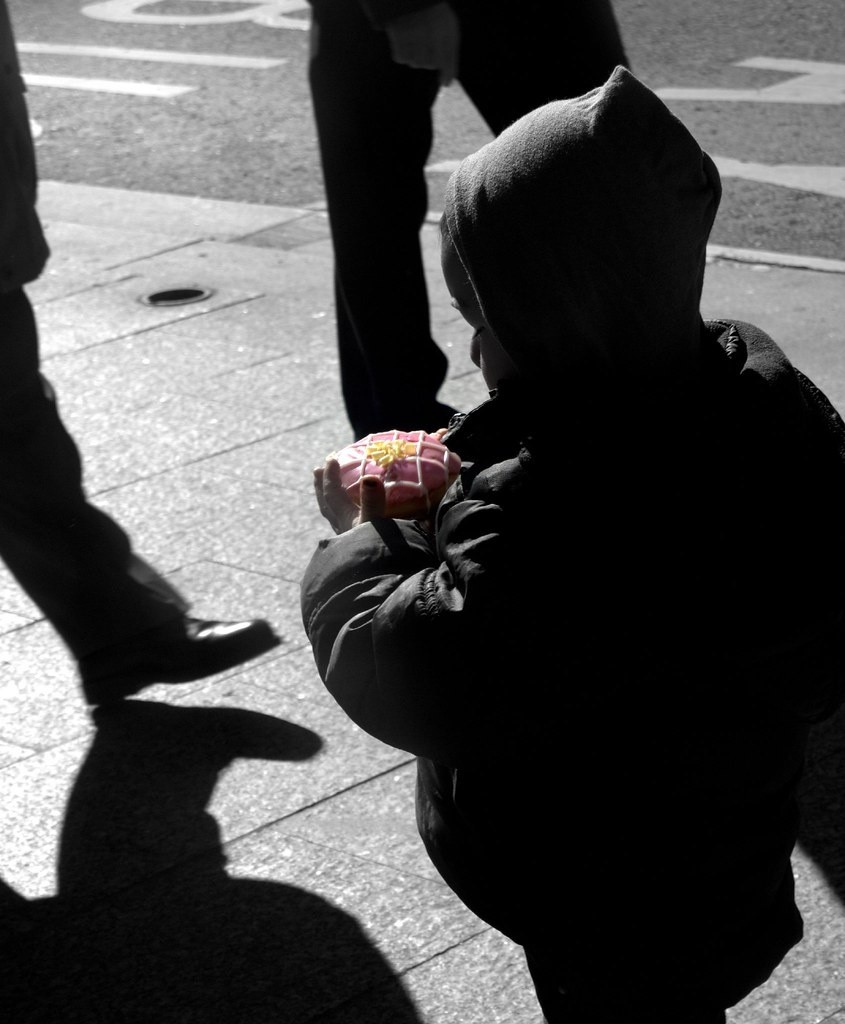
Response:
[324,429,461,521]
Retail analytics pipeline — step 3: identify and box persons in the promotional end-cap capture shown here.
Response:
[299,64,845,1024]
[308,0,631,449]
[0,4,283,707]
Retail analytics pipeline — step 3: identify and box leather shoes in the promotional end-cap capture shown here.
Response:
[79,617,282,705]
